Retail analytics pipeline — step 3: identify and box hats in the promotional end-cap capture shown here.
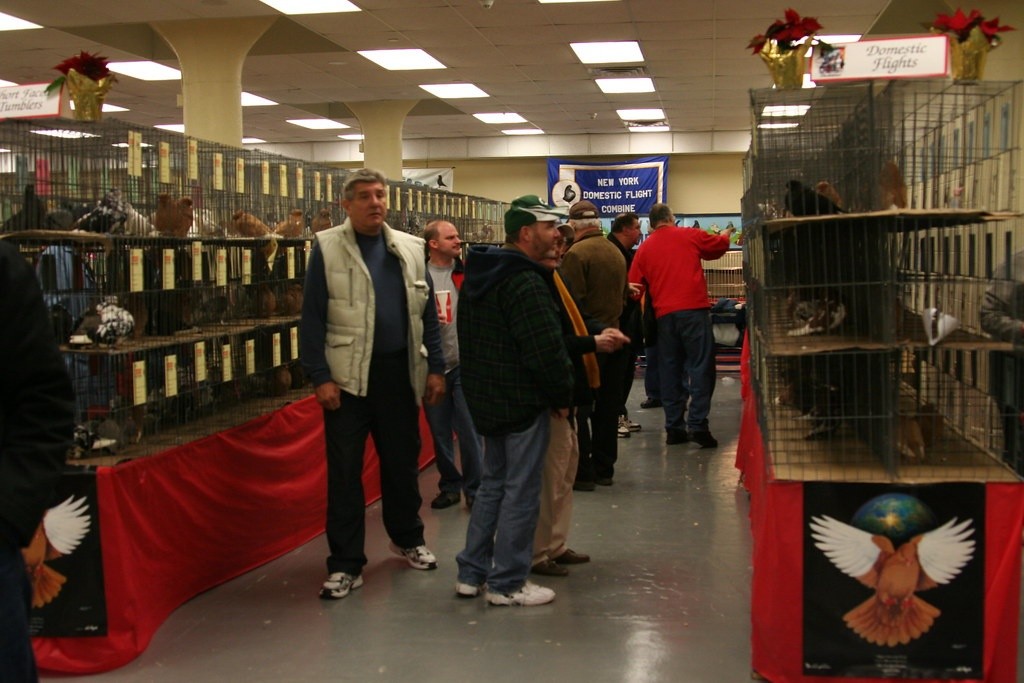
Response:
[569,200,599,219]
[554,219,575,240]
[503,194,570,233]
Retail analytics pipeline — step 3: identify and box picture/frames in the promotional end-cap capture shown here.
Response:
[631,214,743,251]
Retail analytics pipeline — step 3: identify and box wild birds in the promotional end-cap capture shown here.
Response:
[155,192,194,237]
[786,289,846,337]
[96,299,148,345]
[472,223,495,242]
[878,160,907,210]
[438,175,447,188]
[69,186,150,236]
[73,356,305,455]
[783,179,847,217]
[692,220,733,229]
[563,185,576,202]
[274,209,304,238]
[311,209,332,235]
[895,402,943,461]
[232,209,274,237]
[772,381,841,439]
[173,281,303,325]
[405,215,422,236]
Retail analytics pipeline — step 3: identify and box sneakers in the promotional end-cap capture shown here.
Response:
[319,572,364,599]
[622,415,641,432]
[617,415,631,438]
[389,540,438,570]
[487,580,556,606]
[455,582,489,597]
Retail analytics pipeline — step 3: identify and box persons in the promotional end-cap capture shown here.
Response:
[453,195,577,605]
[606,213,645,438]
[420,220,482,511]
[297,168,446,599]
[980,248,1024,480]
[628,202,734,448]
[0,239,74,683]
[550,200,628,492]
[511,194,631,576]
[35,212,127,408]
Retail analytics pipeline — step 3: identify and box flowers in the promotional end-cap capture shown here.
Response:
[745,7,825,54]
[923,7,1017,49]
[51,48,112,82]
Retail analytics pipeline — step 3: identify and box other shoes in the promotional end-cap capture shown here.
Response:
[687,432,717,447]
[572,480,595,491]
[466,496,475,508]
[641,398,662,408]
[666,431,690,444]
[593,476,613,486]
[432,491,461,509]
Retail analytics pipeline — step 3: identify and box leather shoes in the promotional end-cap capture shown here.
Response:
[531,559,568,576]
[552,548,590,564]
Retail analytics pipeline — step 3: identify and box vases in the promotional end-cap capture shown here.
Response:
[762,41,811,89]
[65,69,116,119]
[939,29,991,79]
